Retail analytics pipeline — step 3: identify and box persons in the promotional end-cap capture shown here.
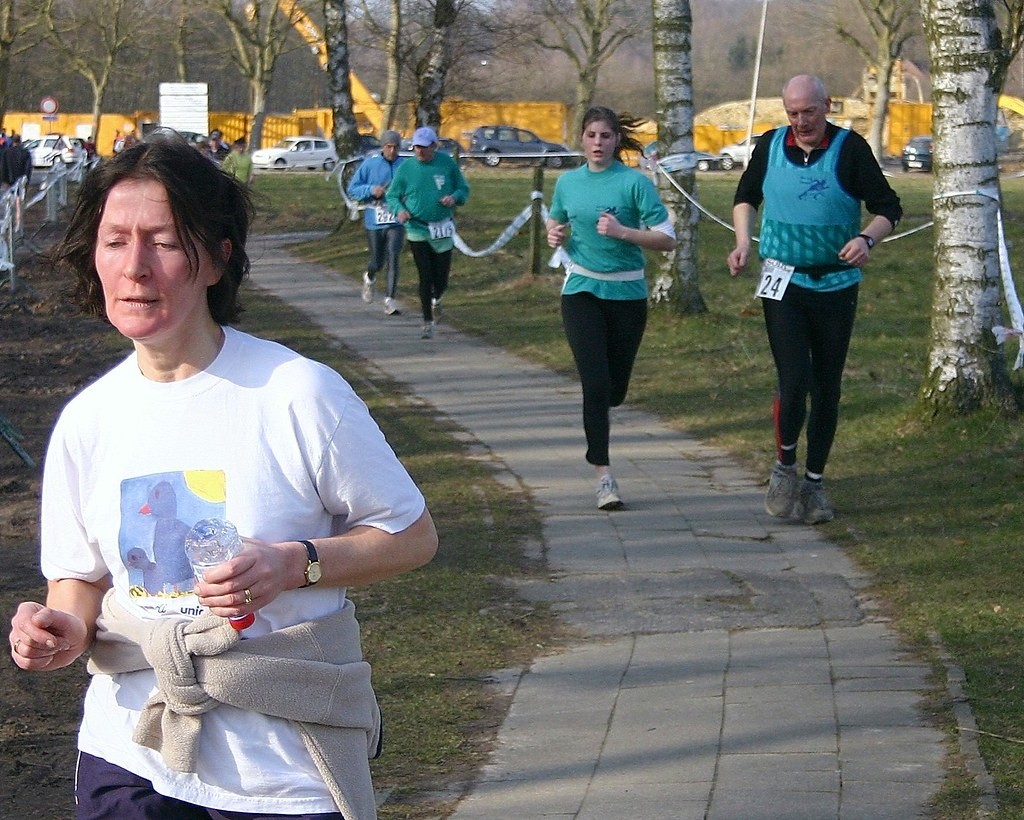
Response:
[0,129,31,232]
[9,135,438,820]
[728,76,903,524]
[546,106,677,509]
[385,127,470,339]
[113,130,136,154]
[347,131,404,315]
[83,137,99,171]
[195,129,254,188]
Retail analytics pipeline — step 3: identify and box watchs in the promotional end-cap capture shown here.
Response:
[297,540,321,589]
[858,234,874,250]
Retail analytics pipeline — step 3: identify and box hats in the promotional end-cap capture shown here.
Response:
[406,127,437,151]
[380,130,401,148]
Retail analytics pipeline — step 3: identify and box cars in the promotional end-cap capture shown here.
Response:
[641,141,719,173]
[359,134,382,151]
[402,136,466,171]
[23,137,88,169]
[252,137,340,171]
[719,134,762,171]
[902,137,934,172]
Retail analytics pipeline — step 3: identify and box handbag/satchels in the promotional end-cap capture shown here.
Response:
[113,138,125,153]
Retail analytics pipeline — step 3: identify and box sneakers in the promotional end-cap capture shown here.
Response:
[764,459,797,518]
[0,261,15,272]
[431,301,442,324]
[362,272,376,303]
[383,300,400,315]
[595,475,623,509]
[421,325,432,339]
[797,479,834,525]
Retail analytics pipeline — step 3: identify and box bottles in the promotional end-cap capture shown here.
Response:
[185,519,256,630]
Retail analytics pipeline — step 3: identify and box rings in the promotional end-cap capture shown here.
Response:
[245,590,251,602]
[857,261,861,265]
[14,640,21,652]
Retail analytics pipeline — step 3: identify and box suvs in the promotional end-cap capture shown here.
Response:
[469,126,571,169]
